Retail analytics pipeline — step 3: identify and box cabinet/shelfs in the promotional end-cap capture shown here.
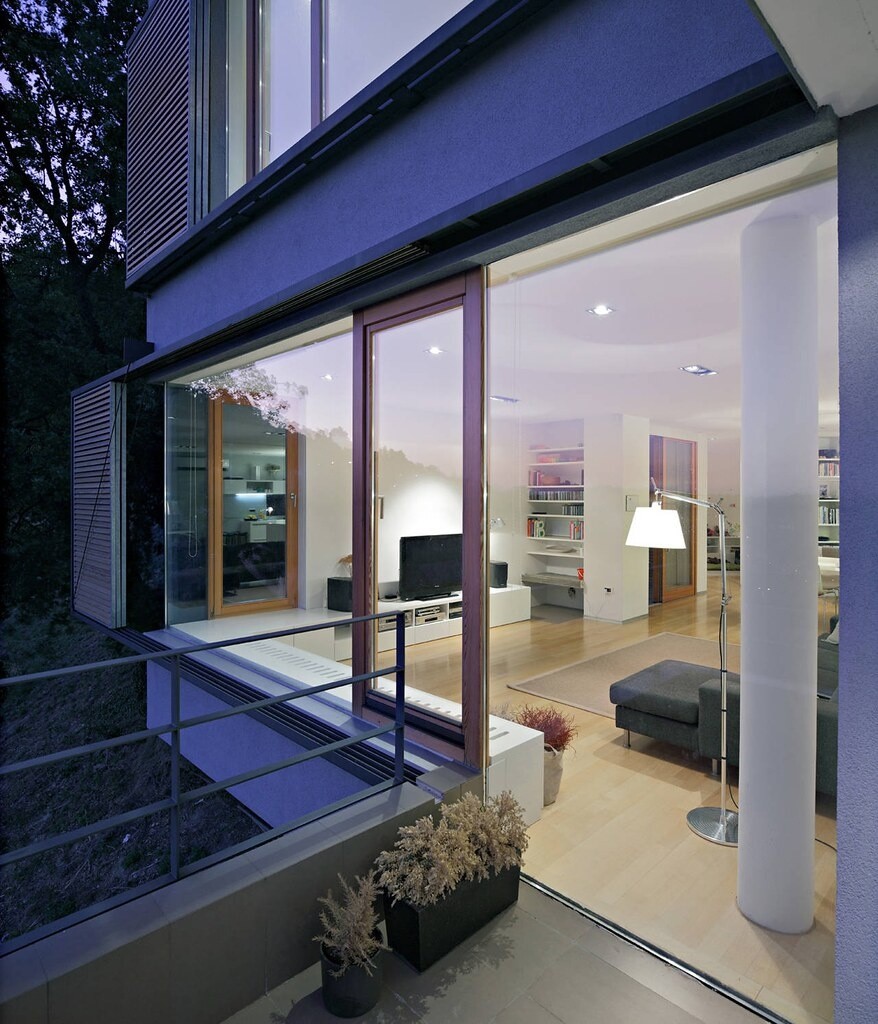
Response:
[818,459,841,549]
[519,412,584,624]
[706,536,740,570]
[293,584,532,665]
[222,477,286,543]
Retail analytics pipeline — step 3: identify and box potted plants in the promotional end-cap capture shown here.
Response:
[364,788,531,976]
[507,703,577,807]
[312,867,393,1021]
[265,463,280,480]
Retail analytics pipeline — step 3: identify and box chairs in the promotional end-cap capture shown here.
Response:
[817,565,839,633]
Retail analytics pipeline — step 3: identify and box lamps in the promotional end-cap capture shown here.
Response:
[624,477,739,849]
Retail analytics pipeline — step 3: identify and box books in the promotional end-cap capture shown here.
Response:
[529,469,544,486]
[818,506,839,524]
[570,521,584,540]
[581,469,584,485]
[820,463,839,476]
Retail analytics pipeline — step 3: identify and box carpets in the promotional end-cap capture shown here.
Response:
[508,631,740,720]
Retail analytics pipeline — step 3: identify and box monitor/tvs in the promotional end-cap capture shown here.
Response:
[399,534,463,601]
[266,494,286,519]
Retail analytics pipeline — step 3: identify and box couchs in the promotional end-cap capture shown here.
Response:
[610,615,837,801]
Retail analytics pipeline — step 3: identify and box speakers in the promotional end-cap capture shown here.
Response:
[489,560,508,588]
[327,577,353,612]
